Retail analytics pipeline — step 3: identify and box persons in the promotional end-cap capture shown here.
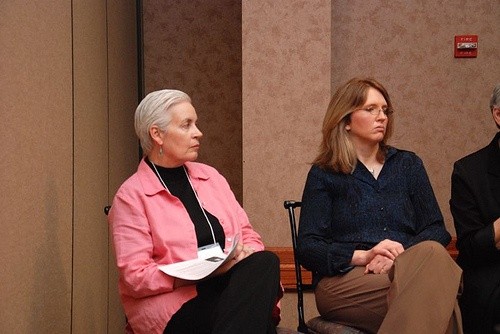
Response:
[449,81,500,333]
[107,88,285,334]
[296,76,465,334]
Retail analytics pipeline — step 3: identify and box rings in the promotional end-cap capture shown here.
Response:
[381,269,388,275]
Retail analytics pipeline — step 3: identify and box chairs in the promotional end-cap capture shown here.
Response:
[284,200,370,334]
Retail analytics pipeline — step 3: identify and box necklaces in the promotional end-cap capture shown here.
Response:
[370,161,380,174]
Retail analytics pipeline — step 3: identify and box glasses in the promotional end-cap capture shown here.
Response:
[358,106,388,114]
